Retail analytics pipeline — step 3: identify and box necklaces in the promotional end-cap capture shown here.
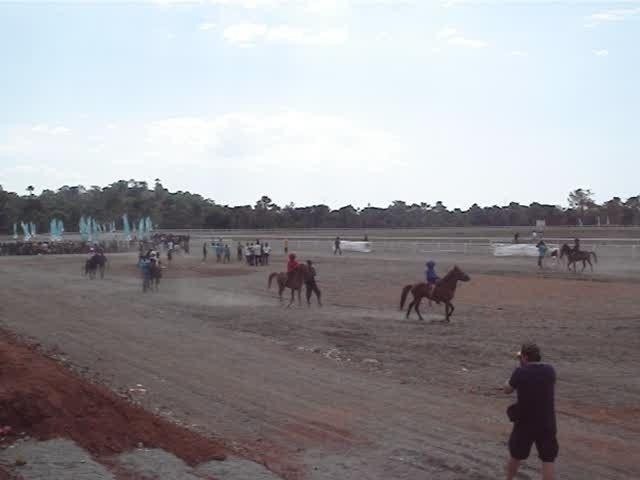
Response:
[550,247,559,266]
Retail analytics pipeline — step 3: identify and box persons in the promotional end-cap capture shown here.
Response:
[502,343,560,480]
[424,260,440,298]
[571,237,581,267]
[302,259,322,306]
[286,253,298,287]
[78,231,273,296]
[332,237,343,256]
[535,239,548,269]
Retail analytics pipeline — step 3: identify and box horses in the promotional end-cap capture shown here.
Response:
[150,263,162,292]
[537,239,566,265]
[561,243,598,272]
[268,263,312,306]
[400,264,471,322]
